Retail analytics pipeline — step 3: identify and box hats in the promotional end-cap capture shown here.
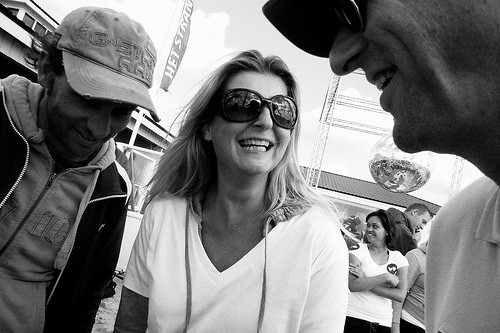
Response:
[53,6,162,123]
[261,0,345,59]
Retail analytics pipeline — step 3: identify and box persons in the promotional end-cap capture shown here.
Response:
[385,202,434,256]
[0,6,160,333]
[261,0,500,333]
[343,208,409,333]
[110,48,349,333]
[391,240,427,333]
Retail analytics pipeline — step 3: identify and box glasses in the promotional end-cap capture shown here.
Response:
[333,0,375,33]
[211,87,299,131]
